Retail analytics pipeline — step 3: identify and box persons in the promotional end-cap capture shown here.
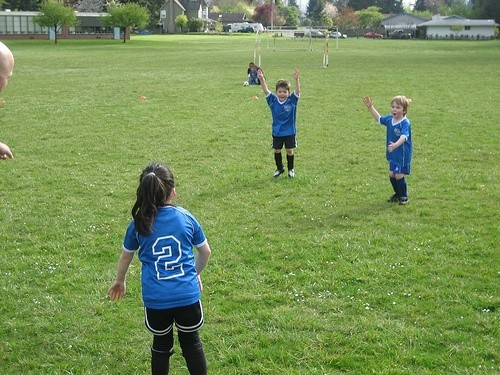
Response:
[247,62,266,85]
[107,162,211,375]
[0,40,14,160]
[257,68,300,178]
[363,95,412,205]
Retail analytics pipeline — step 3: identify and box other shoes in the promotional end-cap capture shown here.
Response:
[388,193,399,202]
[398,198,409,205]
[288,169,295,178]
[273,168,285,177]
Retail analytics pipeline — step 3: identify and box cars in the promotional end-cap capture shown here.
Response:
[293,29,347,39]
[365,32,383,38]
[389,29,411,40]
[237,27,255,33]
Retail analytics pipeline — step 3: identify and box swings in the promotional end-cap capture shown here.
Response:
[271,32,313,53]
